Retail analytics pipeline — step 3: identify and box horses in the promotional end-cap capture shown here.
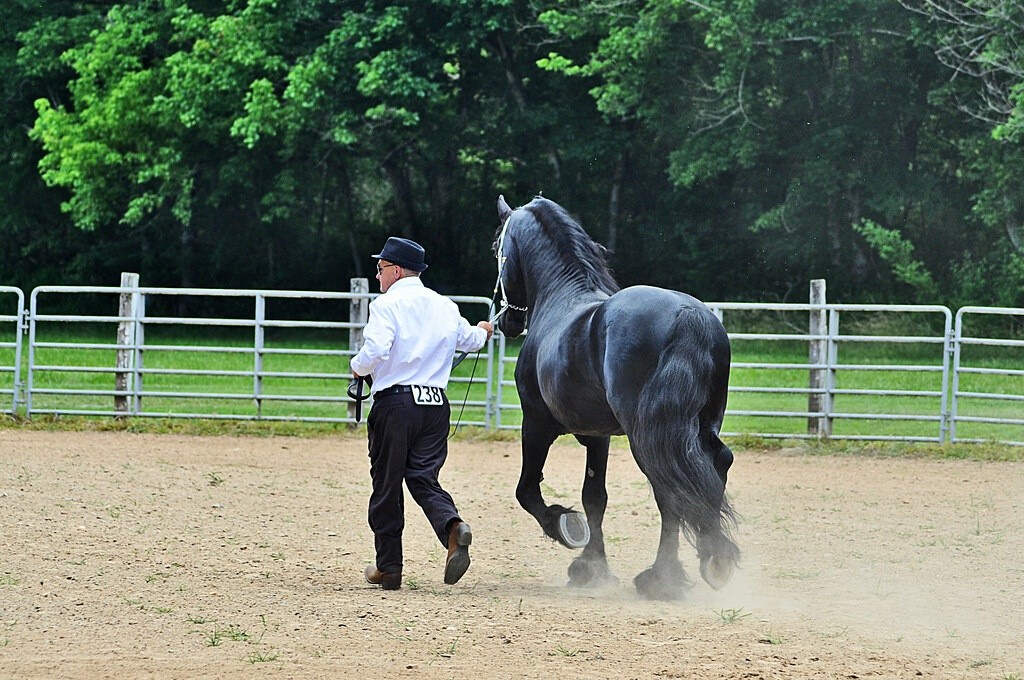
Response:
[494,194,744,601]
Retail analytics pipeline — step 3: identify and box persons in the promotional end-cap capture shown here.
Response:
[350,237,493,591]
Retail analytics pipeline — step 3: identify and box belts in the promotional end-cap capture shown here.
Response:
[375,385,412,400]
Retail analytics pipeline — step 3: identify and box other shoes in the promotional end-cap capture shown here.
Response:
[444,521,472,585]
[365,565,402,590]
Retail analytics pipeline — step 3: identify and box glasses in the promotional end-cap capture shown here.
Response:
[377,264,395,275]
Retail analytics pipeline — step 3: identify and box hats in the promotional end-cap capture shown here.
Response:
[371,236,428,272]
